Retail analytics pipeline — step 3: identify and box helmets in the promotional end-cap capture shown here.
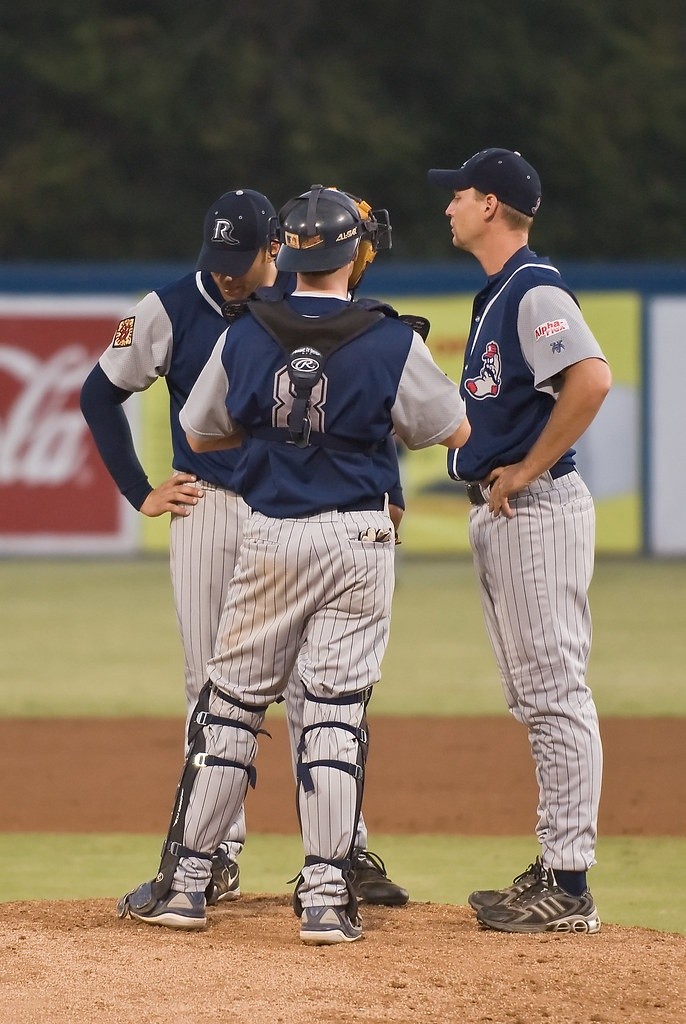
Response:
[274,185,359,272]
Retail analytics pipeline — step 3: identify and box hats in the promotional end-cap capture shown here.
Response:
[426,149,542,218]
[197,190,277,275]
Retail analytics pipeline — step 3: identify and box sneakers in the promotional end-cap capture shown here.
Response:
[477,864,600,933]
[127,875,207,930]
[467,856,543,912]
[207,860,241,906]
[299,906,363,944]
[347,859,409,906]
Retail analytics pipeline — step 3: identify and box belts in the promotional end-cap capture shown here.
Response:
[337,494,385,512]
[465,465,575,505]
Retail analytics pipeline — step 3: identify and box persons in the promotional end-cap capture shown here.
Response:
[77,184,472,945]
[424,148,612,932]
